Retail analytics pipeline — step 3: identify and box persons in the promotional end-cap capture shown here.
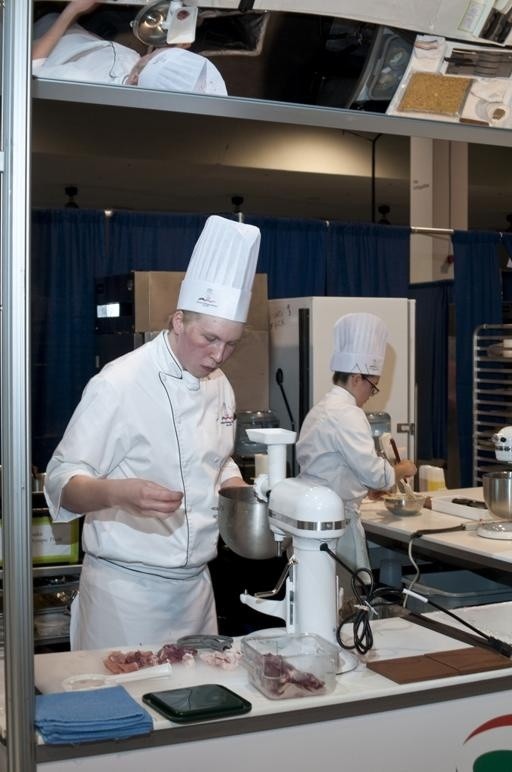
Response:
[296,312,416,603]
[42,216,263,650]
[29,0,229,95]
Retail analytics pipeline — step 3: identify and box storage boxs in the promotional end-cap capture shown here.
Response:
[0,515,80,565]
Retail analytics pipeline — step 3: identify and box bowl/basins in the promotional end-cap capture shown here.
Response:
[217,486,297,561]
[240,632,343,701]
[482,470,512,522]
[383,492,429,516]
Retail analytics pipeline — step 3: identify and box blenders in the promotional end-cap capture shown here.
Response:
[474,423,512,540]
[237,427,372,675]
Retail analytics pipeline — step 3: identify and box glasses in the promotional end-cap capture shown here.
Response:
[360,375,379,395]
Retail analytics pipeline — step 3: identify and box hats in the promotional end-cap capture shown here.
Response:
[136,48,228,100]
[329,312,390,378]
[175,215,260,323]
